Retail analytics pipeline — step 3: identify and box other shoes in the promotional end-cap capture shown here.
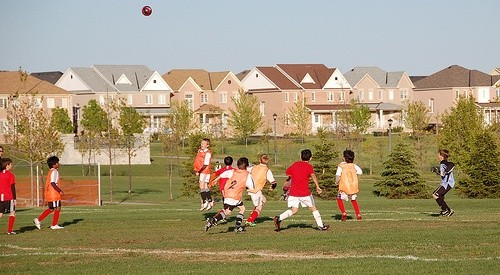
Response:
[357,215,362,220]
[341,213,346,220]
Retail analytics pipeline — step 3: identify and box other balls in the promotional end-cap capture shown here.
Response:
[141,6,151,16]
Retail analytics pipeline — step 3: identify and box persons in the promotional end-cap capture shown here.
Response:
[244,154,277,227]
[0,145,17,235]
[274,149,329,230]
[204,157,256,234]
[431,150,455,217]
[335,150,362,221]
[194,138,216,212]
[33,156,64,230]
[206,156,237,223]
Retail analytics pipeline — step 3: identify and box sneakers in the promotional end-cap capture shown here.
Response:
[439,210,449,216]
[33,218,41,229]
[447,209,455,217]
[207,201,216,210]
[200,200,208,212]
[234,226,246,233]
[274,215,281,231]
[244,221,256,226]
[7,231,16,234]
[318,224,329,231]
[205,218,212,232]
[50,224,64,229]
